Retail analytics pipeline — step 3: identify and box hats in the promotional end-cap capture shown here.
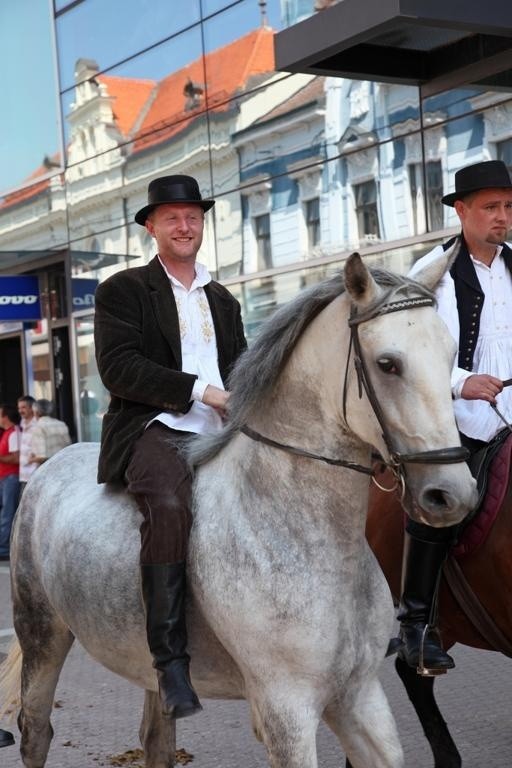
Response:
[134,174,216,226]
[442,159,512,206]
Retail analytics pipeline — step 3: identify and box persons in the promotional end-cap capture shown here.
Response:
[384,160,510,679]
[1,729,17,750]
[25,397,74,476]
[93,173,266,720]
[10,395,39,501]
[0,405,22,561]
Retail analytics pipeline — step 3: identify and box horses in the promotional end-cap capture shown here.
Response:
[1,235,481,768]
[365,423,512,768]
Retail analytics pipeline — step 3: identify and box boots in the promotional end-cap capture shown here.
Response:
[138,558,204,720]
[396,533,457,670]
